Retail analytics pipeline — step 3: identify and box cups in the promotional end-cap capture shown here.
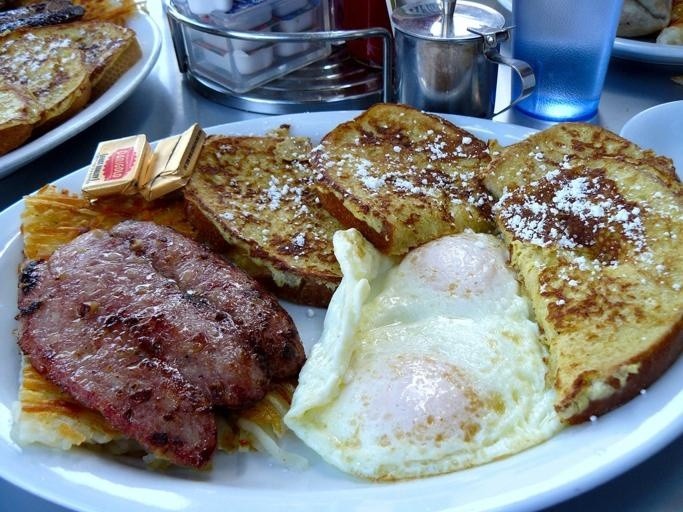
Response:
[392,0,623,122]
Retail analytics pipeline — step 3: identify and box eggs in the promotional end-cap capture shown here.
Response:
[282,227,570,482]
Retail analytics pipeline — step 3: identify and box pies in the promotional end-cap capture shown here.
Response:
[19,183,305,467]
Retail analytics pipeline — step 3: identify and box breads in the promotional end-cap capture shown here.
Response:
[0,0,140,157]
[182,103,683,423]
[617,1,683,45]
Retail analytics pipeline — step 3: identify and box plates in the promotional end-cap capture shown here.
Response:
[0,0,163,179]
[623,96,683,184]
[0,106,683,512]
